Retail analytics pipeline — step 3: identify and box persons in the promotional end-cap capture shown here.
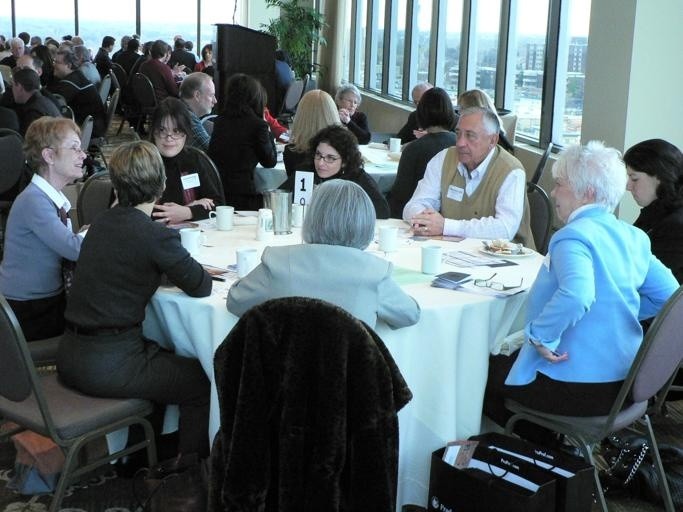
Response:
[388,83,537,250]
[483,139,682,457]
[227,179,421,330]
[1,33,293,223]
[56,140,229,481]
[283,84,394,220]
[0,117,92,341]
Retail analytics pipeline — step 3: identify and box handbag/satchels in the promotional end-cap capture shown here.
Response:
[598,432,682,511]
[12,425,128,495]
[428,442,557,512]
[74,160,105,182]
[469,432,595,512]
[132,461,209,512]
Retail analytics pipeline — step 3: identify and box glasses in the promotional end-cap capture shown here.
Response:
[474,273,524,291]
[154,126,186,139]
[312,151,341,163]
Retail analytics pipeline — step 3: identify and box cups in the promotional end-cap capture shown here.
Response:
[378,225,397,252]
[177,188,306,277]
[388,137,401,153]
[420,244,443,275]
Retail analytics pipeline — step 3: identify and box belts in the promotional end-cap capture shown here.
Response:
[66,317,126,335]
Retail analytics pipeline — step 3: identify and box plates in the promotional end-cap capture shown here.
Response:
[478,244,535,257]
